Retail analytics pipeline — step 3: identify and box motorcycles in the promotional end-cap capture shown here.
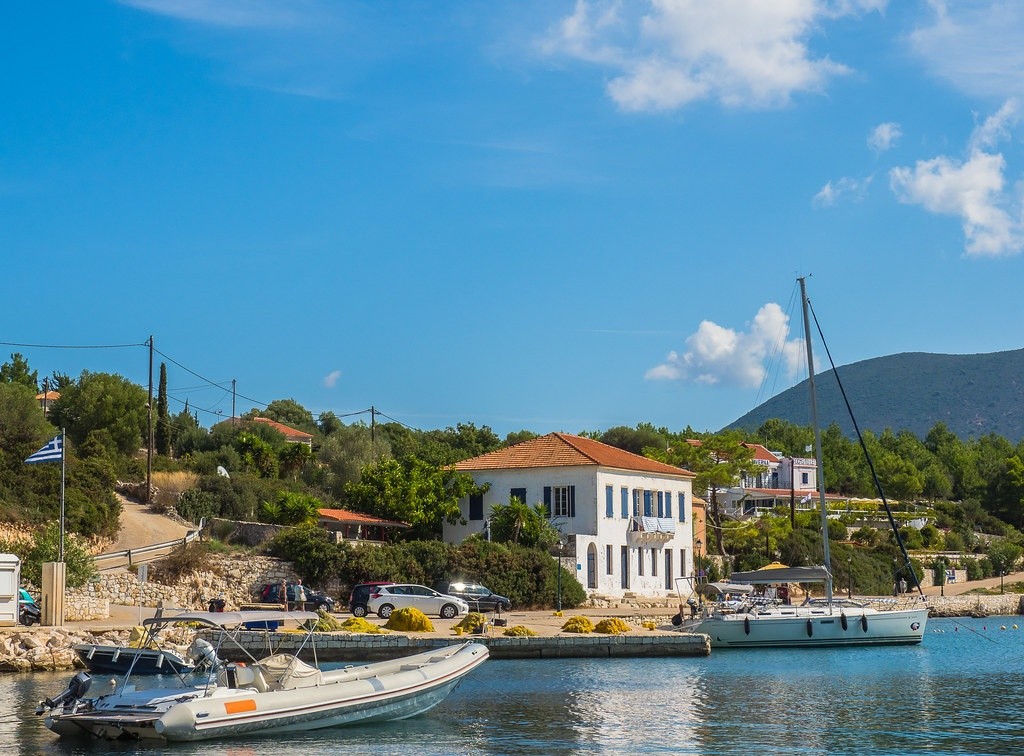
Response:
[17,586,42,627]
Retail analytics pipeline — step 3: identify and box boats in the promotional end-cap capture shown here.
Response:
[34,610,492,748]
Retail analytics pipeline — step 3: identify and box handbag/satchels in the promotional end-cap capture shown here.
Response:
[300,593,307,602]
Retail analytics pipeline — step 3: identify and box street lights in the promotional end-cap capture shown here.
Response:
[1000,558,1004,594]
[554,539,565,613]
[804,554,809,599]
[695,538,704,608]
[939,558,944,596]
[847,555,852,598]
[893,555,899,596]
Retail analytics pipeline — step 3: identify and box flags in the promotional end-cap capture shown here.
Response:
[24,432,63,463]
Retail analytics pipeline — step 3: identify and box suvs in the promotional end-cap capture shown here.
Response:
[366,583,469,619]
[350,580,406,618]
[432,578,512,615]
[251,581,335,614]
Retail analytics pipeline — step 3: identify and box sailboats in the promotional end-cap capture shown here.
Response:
[668,273,931,649]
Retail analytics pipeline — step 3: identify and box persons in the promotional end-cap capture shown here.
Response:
[292,580,307,612]
[279,579,288,611]
